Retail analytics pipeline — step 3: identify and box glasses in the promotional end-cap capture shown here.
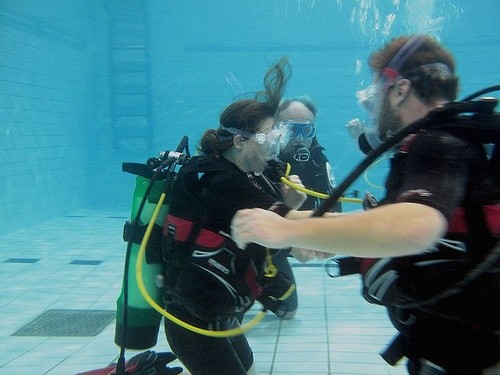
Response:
[217,121,286,153]
[280,117,318,142]
[354,63,450,118]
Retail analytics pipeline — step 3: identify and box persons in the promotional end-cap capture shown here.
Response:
[229,30,500,375]
[242,95,344,320]
[77,55,345,374]
